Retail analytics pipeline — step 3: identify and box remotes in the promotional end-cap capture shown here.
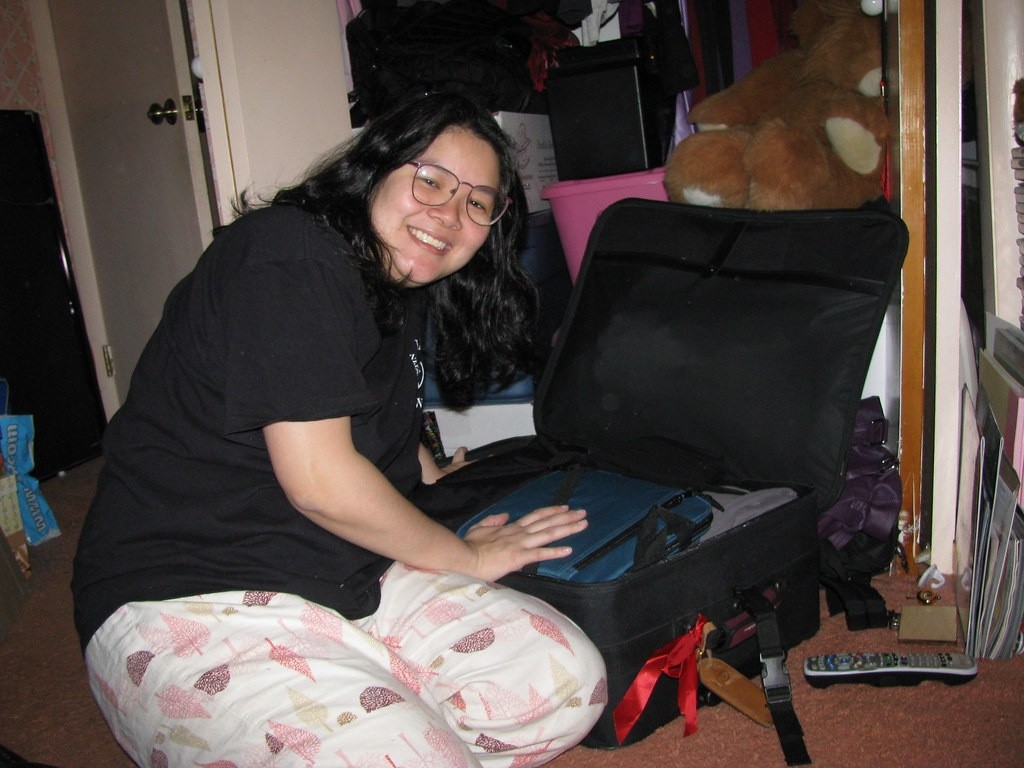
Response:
[801,651,979,689]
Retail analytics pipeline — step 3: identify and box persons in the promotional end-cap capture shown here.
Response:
[68,93,609,768]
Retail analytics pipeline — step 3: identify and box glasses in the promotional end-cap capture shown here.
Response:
[409,160,513,227]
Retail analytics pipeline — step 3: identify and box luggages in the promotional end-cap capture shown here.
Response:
[431,196,910,750]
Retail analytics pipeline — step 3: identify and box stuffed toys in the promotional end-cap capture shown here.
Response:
[1013,77,1024,148]
[665,0,974,212]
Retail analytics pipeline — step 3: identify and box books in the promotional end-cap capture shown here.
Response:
[1011,150,1024,330]
[952,312,1024,659]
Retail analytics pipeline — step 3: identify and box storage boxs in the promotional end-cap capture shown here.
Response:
[541,166,669,290]
[490,110,558,213]
